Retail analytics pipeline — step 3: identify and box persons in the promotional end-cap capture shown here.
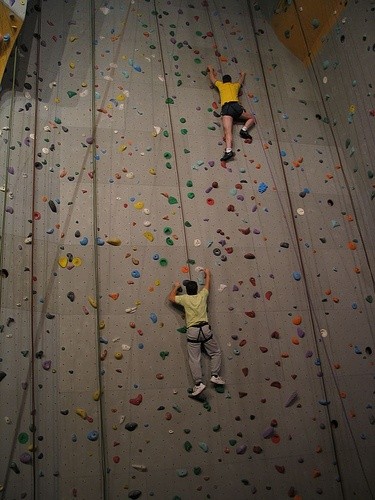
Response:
[207,64,256,161]
[168,267,226,397]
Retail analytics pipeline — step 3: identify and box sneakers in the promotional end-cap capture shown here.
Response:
[192,383,205,396]
[221,150,233,160]
[210,375,225,384]
[240,128,252,139]
